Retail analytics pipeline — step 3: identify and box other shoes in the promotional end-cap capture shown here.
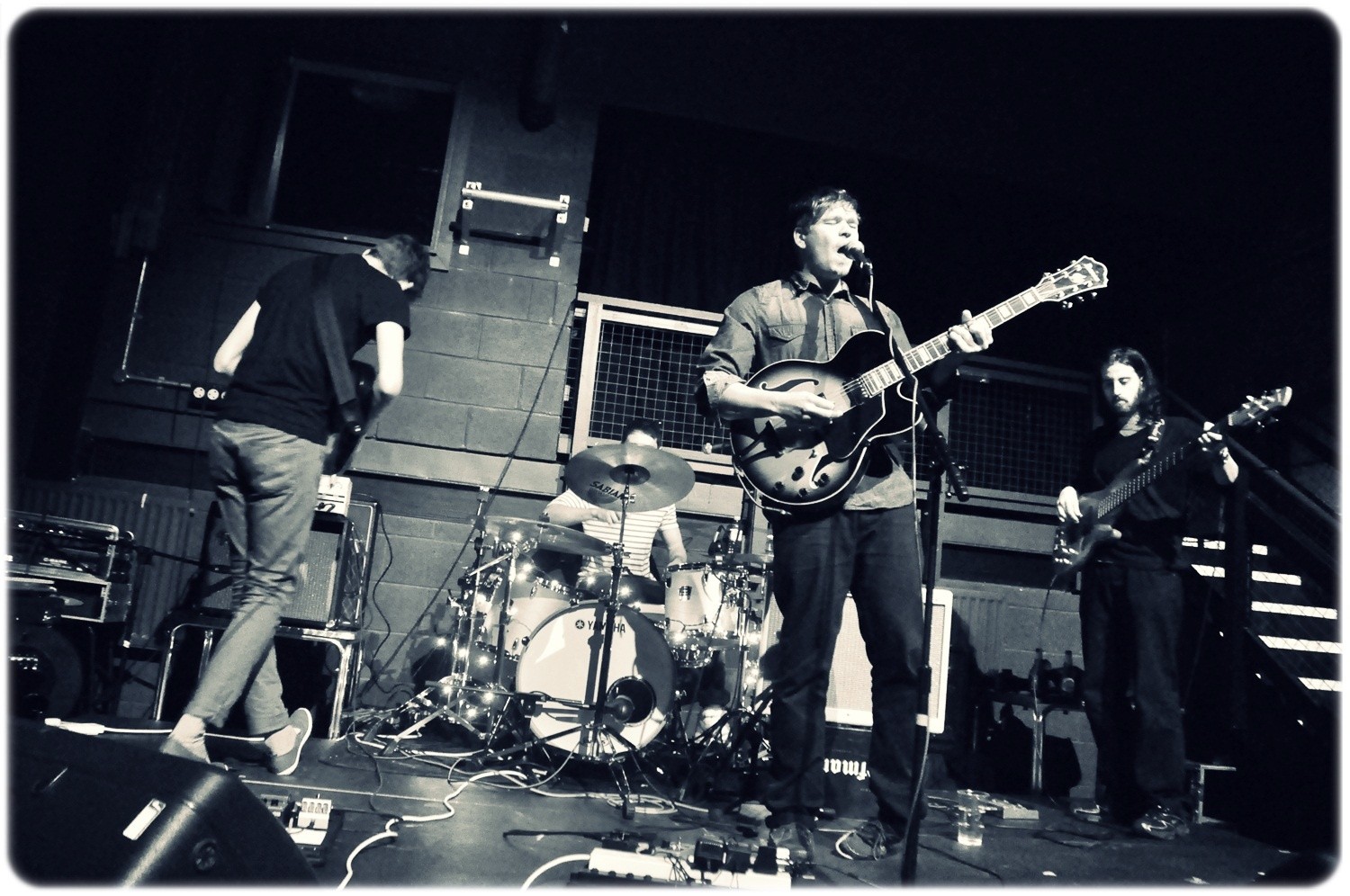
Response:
[268,706,313,775]
[159,737,228,772]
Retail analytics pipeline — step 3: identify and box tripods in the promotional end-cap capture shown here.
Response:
[362,469,770,820]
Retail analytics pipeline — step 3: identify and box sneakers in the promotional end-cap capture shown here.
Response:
[1069,796,1112,828]
[834,816,897,863]
[764,821,816,870]
[1132,802,1189,838]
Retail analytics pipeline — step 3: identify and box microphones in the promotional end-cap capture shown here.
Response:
[702,442,729,454]
[708,525,722,556]
[844,240,875,276]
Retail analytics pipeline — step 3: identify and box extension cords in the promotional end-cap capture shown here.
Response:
[586,846,792,891]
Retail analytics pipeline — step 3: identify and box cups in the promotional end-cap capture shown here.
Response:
[955,789,991,847]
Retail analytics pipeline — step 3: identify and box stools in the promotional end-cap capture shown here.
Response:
[153,622,370,741]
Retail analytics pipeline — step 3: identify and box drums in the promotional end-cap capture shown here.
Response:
[479,568,587,665]
[663,559,764,651]
[514,599,677,763]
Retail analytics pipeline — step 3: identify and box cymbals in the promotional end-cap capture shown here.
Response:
[465,514,616,558]
[564,443,696,513]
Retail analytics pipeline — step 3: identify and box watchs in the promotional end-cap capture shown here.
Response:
[1219,446,1229,467]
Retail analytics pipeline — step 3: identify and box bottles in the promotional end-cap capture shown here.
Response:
[1064,650,1073,667]
[1028,648,1046,678]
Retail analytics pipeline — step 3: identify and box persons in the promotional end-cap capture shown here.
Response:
[157,234,429,772]
[1054,346,1240,841]
[541,420,690,582]
[697,186,994,866]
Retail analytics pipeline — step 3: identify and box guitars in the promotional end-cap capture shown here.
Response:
[318,358,377,477]
[727,255,1110,515]
[1053,385,1293,581]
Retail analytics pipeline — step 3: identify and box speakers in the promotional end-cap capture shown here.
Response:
[193,500,380,630]
[755,586,953,735]
[8,716,320,887]
